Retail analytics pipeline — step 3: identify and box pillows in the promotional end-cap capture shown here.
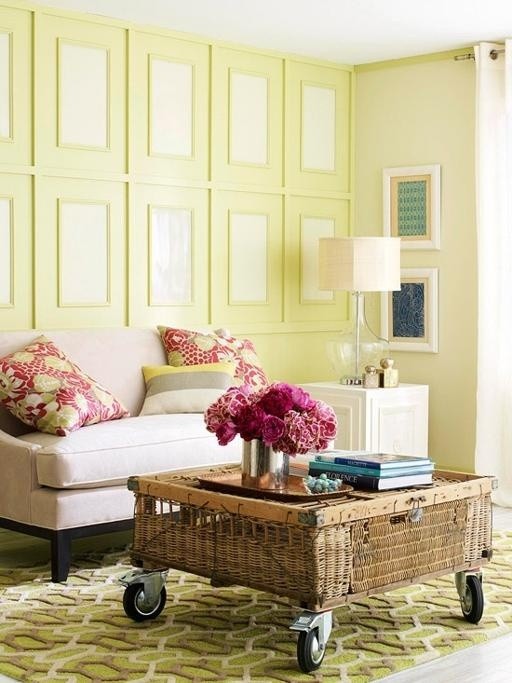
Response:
[137,323,268,419]
[3,332,130,437]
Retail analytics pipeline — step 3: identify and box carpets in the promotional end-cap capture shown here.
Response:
[1,522,511,681]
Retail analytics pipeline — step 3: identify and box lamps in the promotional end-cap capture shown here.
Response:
[317,234,403,387]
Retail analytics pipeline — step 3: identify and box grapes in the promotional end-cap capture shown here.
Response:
[304,472,342,494]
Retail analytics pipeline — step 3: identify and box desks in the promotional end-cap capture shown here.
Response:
[308,385,433,467]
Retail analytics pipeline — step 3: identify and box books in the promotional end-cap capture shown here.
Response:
[286,442,438,492]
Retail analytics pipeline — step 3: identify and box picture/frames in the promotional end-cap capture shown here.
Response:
[383,266,442,356]
[382,165,442,253]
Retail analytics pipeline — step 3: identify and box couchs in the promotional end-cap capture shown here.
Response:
[1,322,285,585]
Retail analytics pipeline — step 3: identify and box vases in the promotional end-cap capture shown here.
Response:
[241,434,274,479]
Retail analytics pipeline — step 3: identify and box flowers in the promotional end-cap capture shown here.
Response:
[204,383,337,457]
